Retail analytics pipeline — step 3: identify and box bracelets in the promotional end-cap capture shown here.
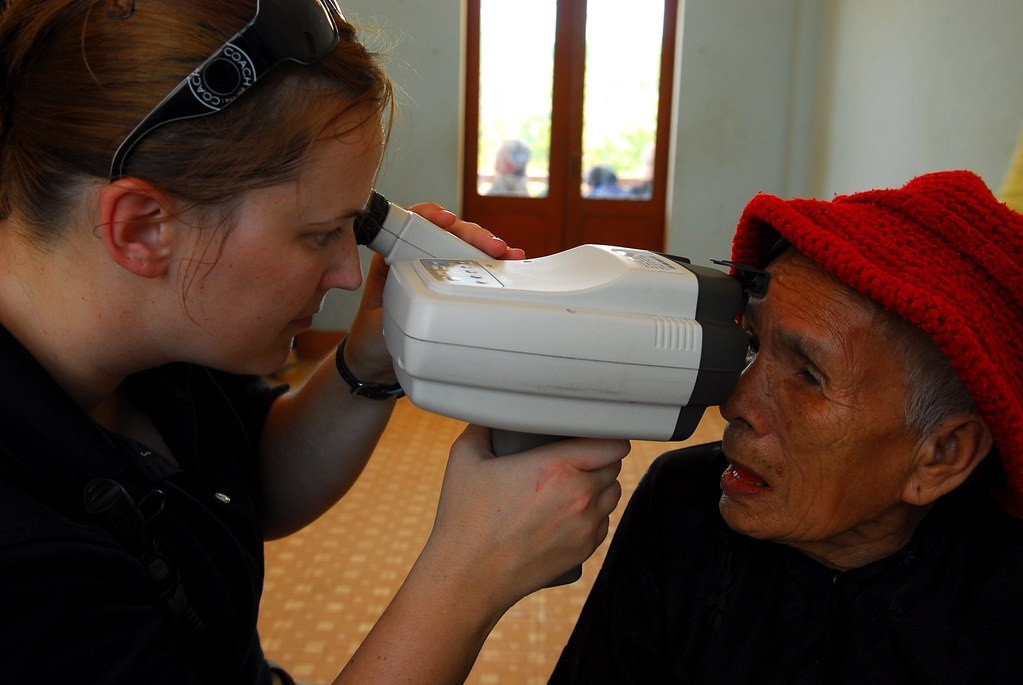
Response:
[335,332,406,400]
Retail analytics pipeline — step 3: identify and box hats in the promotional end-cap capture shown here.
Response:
[730,170,1023,521]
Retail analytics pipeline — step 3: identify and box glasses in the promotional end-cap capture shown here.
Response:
[110,0,346,184]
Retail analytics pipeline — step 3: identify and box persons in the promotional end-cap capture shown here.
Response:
[0,0,633,685]
[537,170,1023,685]
[587,167,627,197]
[486,140,531,196]
